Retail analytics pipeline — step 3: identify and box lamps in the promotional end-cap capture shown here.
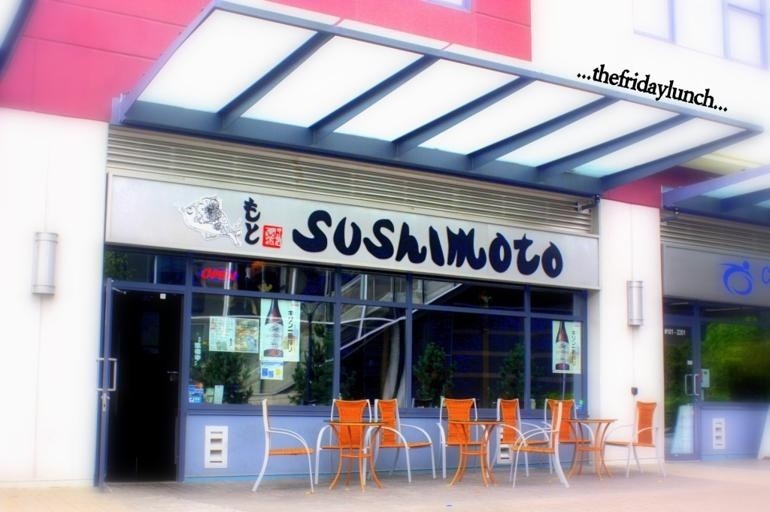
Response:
[626,280,643,326]
[32,231,58,295]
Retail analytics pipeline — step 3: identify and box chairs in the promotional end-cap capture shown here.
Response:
[251,397,667,495]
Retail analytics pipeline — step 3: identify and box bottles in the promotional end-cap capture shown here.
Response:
[264,299,283,357]
[555,320,570,370]
